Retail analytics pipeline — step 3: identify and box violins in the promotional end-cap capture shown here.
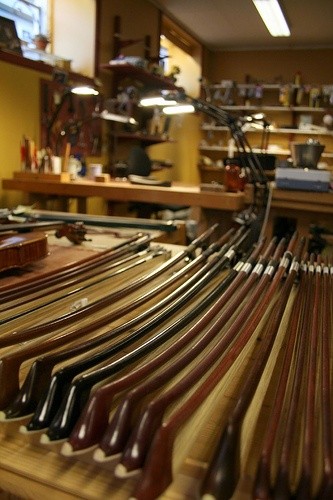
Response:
[0,220,93,272]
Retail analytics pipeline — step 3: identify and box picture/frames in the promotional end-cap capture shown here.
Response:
[0,16,24,57]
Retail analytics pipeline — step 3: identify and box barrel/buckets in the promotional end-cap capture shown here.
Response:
[294,144,325,169]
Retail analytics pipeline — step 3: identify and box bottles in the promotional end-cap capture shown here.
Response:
[224,160,245,192]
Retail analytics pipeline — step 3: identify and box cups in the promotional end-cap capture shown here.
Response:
[52,156,61,173]
[89,163,103,177]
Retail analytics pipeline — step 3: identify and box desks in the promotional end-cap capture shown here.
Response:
[1,177,246,245]
[0,233,325,500]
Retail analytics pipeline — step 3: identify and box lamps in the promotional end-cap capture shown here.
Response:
[47,81,272,228]
[252,0,293,39]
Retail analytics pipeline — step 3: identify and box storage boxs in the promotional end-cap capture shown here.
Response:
[224,165,245,193]
[236,152,276,170]
[298,113,314,130]
[21,48,73,72]
[276,158,331,194]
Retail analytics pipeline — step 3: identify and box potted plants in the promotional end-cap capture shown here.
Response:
[33,33,52,51]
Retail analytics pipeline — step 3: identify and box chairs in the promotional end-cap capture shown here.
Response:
[125,145,191,219]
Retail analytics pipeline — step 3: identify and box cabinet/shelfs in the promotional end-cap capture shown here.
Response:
[199,79,333,185]
[101,14,181,218]
